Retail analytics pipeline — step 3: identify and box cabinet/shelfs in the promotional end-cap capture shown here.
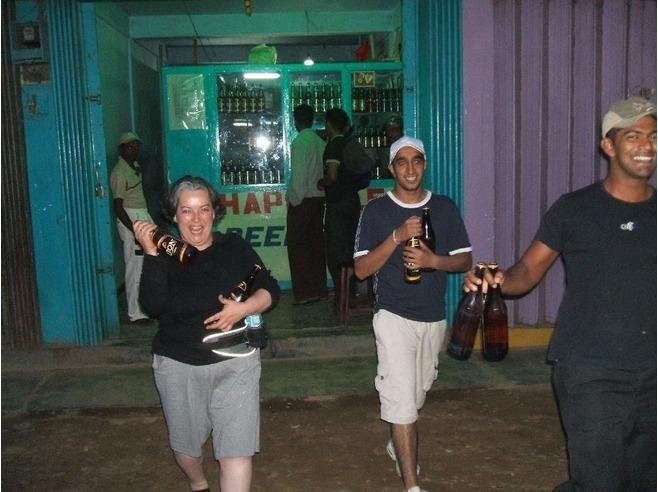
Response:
[221,108,403,188]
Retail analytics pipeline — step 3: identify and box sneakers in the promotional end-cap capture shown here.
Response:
[386,441,420,478]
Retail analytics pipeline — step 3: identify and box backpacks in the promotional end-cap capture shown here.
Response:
[342,136,374,191]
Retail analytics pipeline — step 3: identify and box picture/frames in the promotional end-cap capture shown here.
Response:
[354,71,375,87]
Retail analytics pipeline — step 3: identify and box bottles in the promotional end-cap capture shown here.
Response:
[217,264,262,322]
[374,156,383,180]
[291,82,342,113]
[351,124,388,149]
[421,206,436,272]
[353,88,402,113]
[447,263,487,361]
[134,220,196,265]
[404,236,422,284]
[483,263,508,362]
[220,158,282,184]
[217,74,265,114]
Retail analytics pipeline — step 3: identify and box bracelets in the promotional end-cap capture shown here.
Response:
[392,229,400,246]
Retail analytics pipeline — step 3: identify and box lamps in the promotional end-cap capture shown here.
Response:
[303,10,315,66]
[243,41,280,80]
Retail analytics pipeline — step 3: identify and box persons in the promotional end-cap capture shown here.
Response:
[376,114,403,180]
[464,98,657,492]
[352,137,473,491]
[285,104,329,305]
[110,132,155,325]
[317,106,371,302]
[133,174,281,492]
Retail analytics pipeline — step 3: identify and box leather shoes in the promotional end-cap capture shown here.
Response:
[127,317,155,327]
[291,294,321,306]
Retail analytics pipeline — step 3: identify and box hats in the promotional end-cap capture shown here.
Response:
[116,131,145,148]
[389,135,427,165]
[598,96,657,139]
